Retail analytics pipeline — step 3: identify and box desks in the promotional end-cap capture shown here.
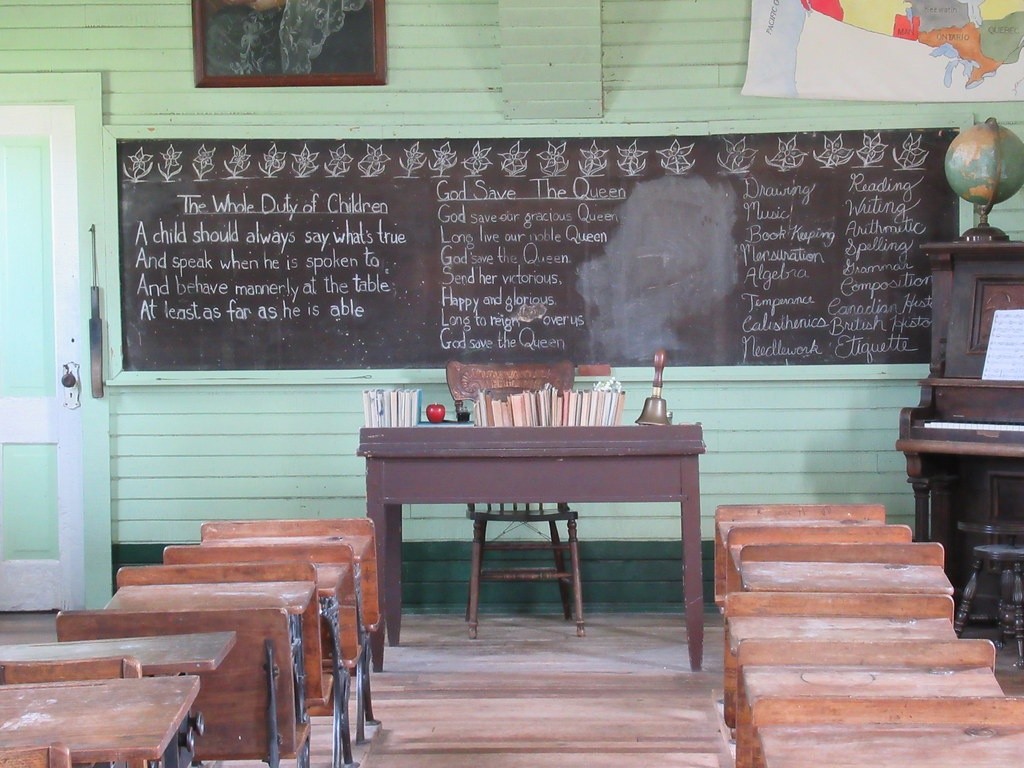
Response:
[356,424,706,672]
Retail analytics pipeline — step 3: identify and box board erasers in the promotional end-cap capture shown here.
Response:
[576,364,612,376]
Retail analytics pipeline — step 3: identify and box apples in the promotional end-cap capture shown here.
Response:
[426,404,446,422]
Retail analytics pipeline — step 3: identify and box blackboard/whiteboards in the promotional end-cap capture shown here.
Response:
[192,1,387,86]
[104,110,974,384]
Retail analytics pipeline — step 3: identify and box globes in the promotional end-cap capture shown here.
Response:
[944,115,1024,242]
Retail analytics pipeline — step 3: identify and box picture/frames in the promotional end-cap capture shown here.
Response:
[192,0,388,87]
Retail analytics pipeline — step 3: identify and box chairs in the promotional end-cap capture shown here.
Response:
[0,517,383,768]
[446,360,586,639]
[714,503,1024,768]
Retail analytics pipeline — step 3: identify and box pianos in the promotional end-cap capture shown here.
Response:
[894,239,1024,593]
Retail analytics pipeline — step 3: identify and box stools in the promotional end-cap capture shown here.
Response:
[954,545,1024,668]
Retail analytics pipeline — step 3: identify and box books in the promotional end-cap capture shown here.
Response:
[472,383,625,427]
[363,388,422,428]
